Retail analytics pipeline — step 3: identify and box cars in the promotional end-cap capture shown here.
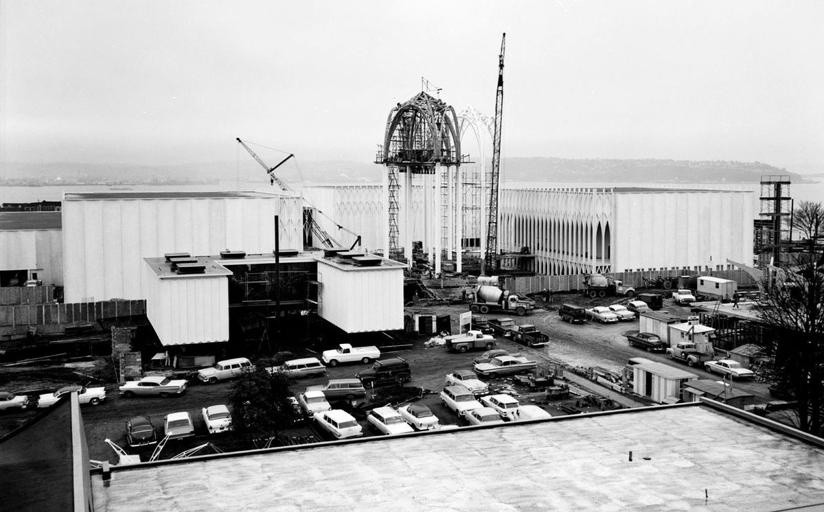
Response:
[36,384,107,410]
[118,376,189,397]
[264,317,551,441]
[201,402,234,435]
[161,411,195,441]
[705,358,757,382]
[197,356,255,386]
[628,332,666,353]
[124,413,158,447]
[584,300,654,323]
[0,392,32,413]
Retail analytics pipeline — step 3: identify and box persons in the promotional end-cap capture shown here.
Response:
[732,289,740,309]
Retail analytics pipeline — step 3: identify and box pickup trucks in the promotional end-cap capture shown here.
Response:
[672,288,696,305]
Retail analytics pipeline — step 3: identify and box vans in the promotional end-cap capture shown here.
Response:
[559,303,587,323]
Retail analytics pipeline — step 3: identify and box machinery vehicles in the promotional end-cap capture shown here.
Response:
[466,285,535,315]
[583,273,636,299]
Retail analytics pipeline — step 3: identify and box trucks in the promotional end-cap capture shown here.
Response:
[666,341,712,366]
[24,279,42,288]
